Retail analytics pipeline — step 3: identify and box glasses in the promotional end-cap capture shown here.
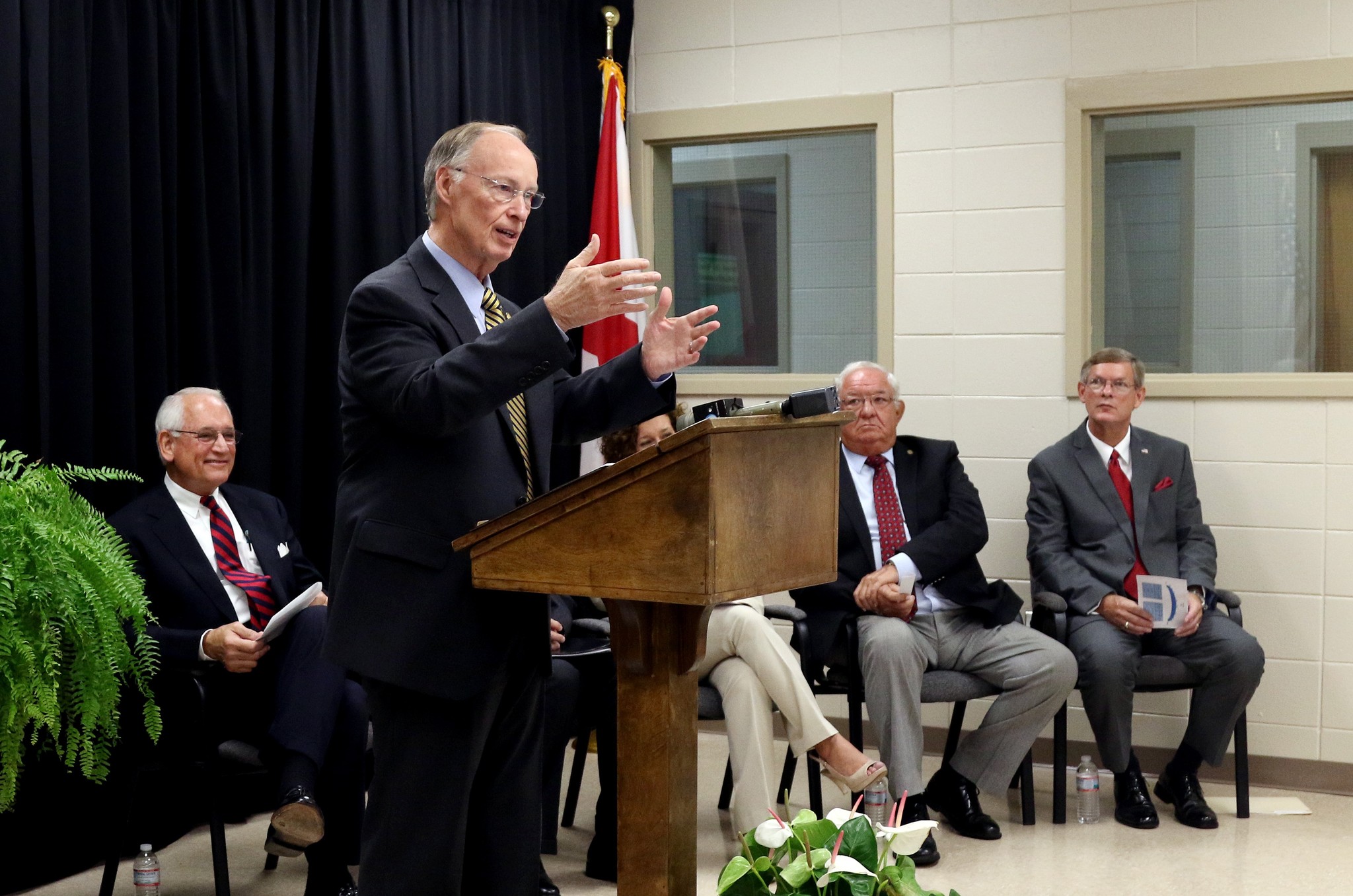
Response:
[456,167,547,209]
[839,392,896,410]
[173,427,244,445]
[1086,376,1136,392]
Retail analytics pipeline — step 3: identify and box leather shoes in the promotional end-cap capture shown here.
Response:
[1115,771,1160,830]
[538,856,561,896]
[923,766,1003,840]
[892,799,940,868]
[339,882,366,896]
[584,834,618,882]
[265,784,324,858]
[1153,762,1219,829]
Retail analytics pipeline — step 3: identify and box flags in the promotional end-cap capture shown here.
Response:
[567,58,654,485]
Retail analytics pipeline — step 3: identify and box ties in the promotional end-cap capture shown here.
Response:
[1108,449,1150,603]
[481,287,537,502]
[199,495,278,632]
[863,453,919,623]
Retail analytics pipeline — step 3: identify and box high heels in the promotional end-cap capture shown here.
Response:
[808,746,889,795]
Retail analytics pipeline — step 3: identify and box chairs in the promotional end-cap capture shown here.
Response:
[94,578,372,895]
[558,585,817,861]
[773,575,1040,844]
[1018,545,1264,829]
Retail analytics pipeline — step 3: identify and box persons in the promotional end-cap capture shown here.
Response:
[598,399,890,852]
[326,114,722,896]
[787,358,1081,871]
[99,380,371,896]
[525,588,621,896]
[1024,343,1269,834]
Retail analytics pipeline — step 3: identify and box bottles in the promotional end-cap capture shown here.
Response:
[865,778,888,845]
[134,843,160,896]
[1077,755,1101,823]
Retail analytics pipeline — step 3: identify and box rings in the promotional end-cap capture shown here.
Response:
[1124,621,1129,629]
[689,341,693,352]
[1196,623,1200,629]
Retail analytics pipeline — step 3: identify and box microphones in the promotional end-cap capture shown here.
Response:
[735,386,841,420]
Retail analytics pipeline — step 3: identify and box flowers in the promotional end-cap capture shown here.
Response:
[713,783,962,896]
